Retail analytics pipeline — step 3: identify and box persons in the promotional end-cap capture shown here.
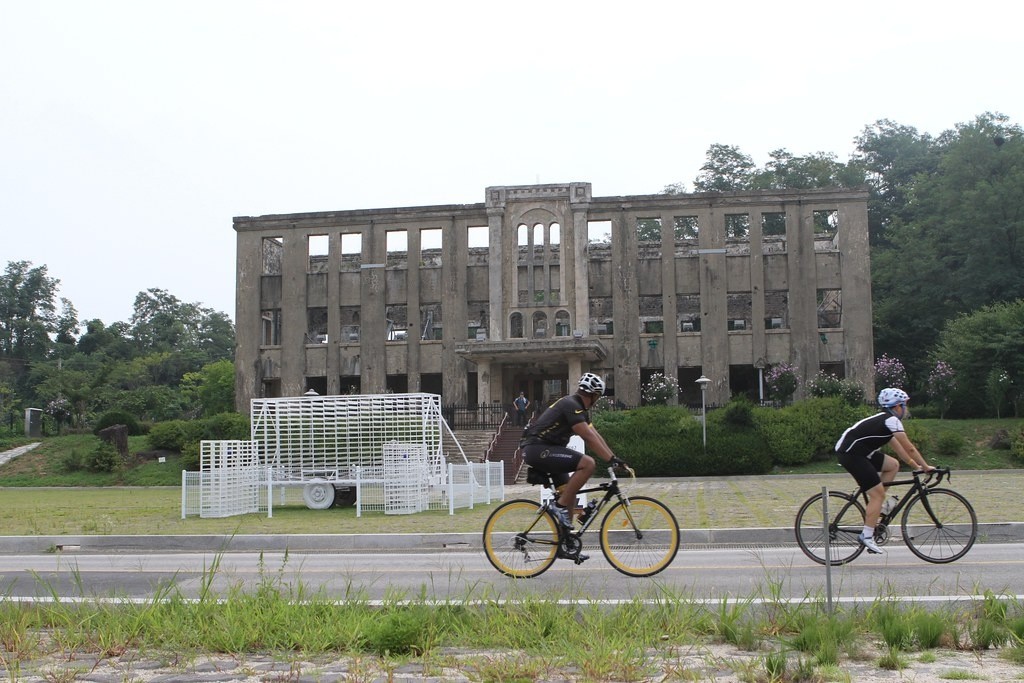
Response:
[834,387,937,554]
[513,391,531,429]
[519,372,633,560]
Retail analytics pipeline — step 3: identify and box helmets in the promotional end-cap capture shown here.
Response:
[578,372,605,395]
[878,388,910,408]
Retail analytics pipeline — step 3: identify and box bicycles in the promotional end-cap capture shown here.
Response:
[794,465,978,567]
[481,462,681,577]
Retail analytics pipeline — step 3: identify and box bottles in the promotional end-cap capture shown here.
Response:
[577,499,598,525]
[879,495,900,516]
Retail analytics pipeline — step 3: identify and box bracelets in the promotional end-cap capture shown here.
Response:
[907,459,913,465]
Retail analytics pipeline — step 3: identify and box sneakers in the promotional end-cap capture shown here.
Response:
[881,504,888,516]
[548,501,574,529]
[562,546,589,560]
[857,532,883,554]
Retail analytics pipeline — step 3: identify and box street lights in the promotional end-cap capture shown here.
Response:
[758,366,765,407]
[694,374,713,446]
